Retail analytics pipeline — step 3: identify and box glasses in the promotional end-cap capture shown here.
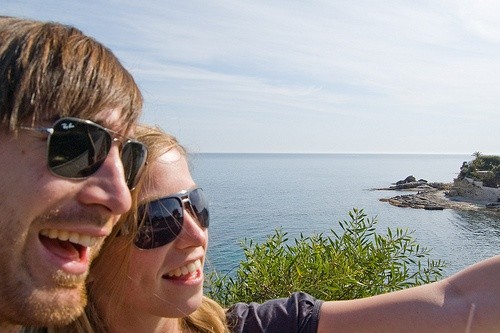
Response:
[17,117,146,193]
[109,187,210,249]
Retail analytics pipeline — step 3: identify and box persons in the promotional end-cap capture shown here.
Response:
[171,208,182,238]
[80,126,106,176]
[27,124,500,330]
[192,205,208,227]
[0,14,143,332]
